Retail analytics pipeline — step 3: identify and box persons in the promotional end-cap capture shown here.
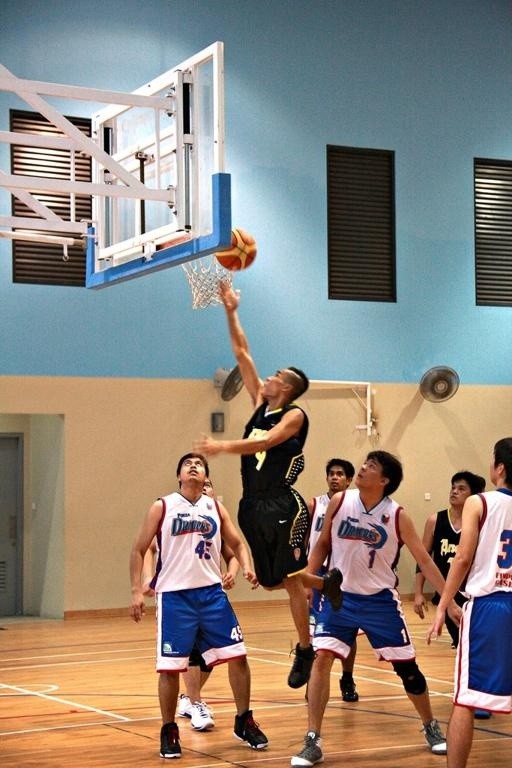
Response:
[192,281,344,688]
[142,477,240,732]
[426,435,512,768]
[305,457,358,703]
[289,450,464,768]
[414,469,492,720]
[129,451,269,760]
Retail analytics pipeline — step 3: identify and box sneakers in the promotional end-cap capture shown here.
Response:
[189,700,215,731]
[339,676,358,702]
[158,720,182,760]
[178,695,214,719]
[287,642,315,689]
[291,730,324,767]
[422,719,447,755]
[232,710,270,749]
[320,567,345,611]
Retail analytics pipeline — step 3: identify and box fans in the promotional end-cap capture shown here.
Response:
[215,365,245,401]
[419,365,460,404]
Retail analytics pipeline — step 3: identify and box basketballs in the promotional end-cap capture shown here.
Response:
[215,227,257,271]
